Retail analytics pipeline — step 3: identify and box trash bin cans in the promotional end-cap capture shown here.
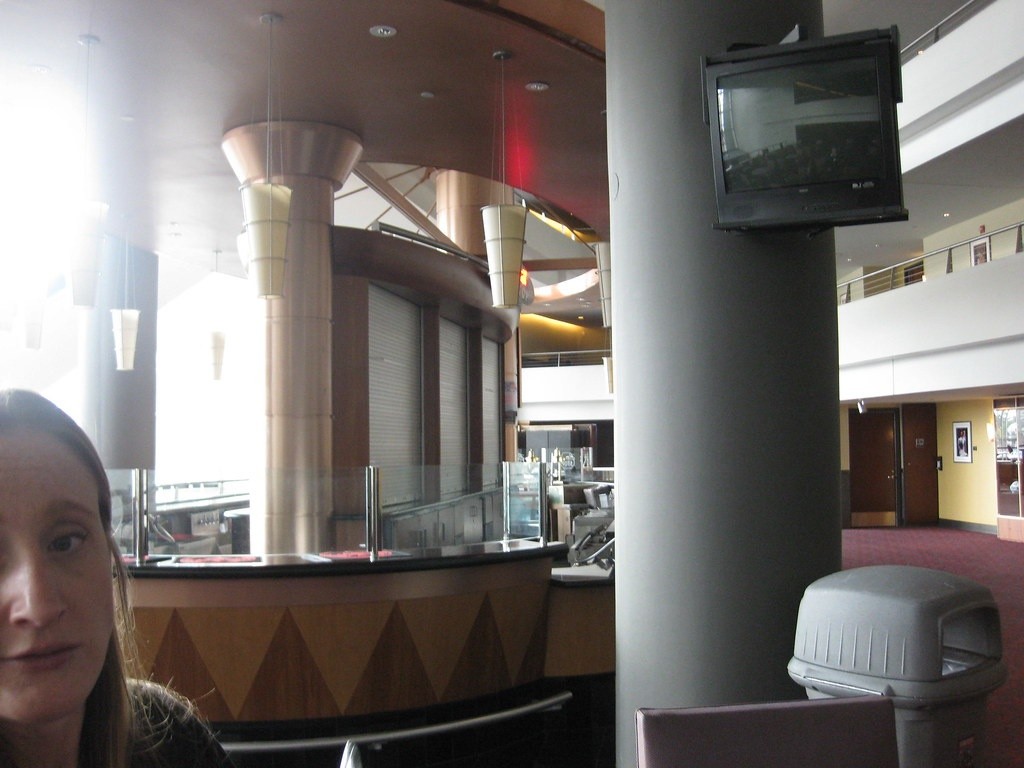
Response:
[788,565,1008,768]
[224,507,250,555]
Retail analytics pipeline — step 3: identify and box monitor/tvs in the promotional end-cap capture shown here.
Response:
[703,40,903,226]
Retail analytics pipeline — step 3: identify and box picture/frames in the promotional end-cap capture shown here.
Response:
[952,421,974,464]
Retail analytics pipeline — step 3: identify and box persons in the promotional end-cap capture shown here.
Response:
[0,388,235,768]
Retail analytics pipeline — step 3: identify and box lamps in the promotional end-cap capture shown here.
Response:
[108,116,139,370]
[478,50,527,308]
[239,13,293,299]
[595,242,612,328]
[206,248,223,380]
[936,456,942,471]
[602,328,613,394]
[857,399,867,414]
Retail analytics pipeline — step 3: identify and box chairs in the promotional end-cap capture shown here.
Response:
[634,695,899,768]
